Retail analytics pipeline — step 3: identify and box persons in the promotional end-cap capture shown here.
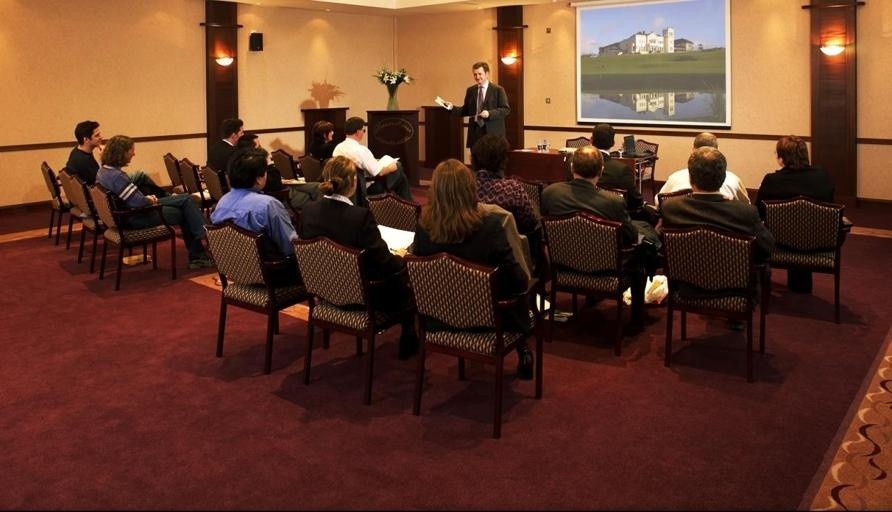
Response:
[441,62,513,179]
[207,117,299,285]
[307,117,414,203]
[540,124,661,336]
[298,155,419,361]
[754,135,835,293]
[653,132,775,332]
[412,134,552,381]
[66,122,216,270]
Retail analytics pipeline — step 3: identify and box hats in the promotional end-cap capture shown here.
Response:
[345,117,370,130]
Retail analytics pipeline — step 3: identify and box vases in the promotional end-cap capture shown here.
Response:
[386,94,399,111]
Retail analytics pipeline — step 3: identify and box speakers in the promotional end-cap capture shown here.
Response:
[249,33,263,51]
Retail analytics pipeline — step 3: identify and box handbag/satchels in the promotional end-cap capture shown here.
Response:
[622,150,653,157]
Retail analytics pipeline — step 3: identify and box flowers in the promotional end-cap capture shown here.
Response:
[372,63,417,97]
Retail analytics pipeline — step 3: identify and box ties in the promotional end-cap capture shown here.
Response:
[477,86,485,128]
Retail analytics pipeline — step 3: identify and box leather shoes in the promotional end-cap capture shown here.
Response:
[189,253,215,268]
[194,224,206,240]
[517,344,534,380]
[399,329,418,359]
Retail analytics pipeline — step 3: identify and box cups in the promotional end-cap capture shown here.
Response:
[537,144,551,153]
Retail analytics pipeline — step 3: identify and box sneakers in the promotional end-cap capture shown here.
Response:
[730,317,746,331]
